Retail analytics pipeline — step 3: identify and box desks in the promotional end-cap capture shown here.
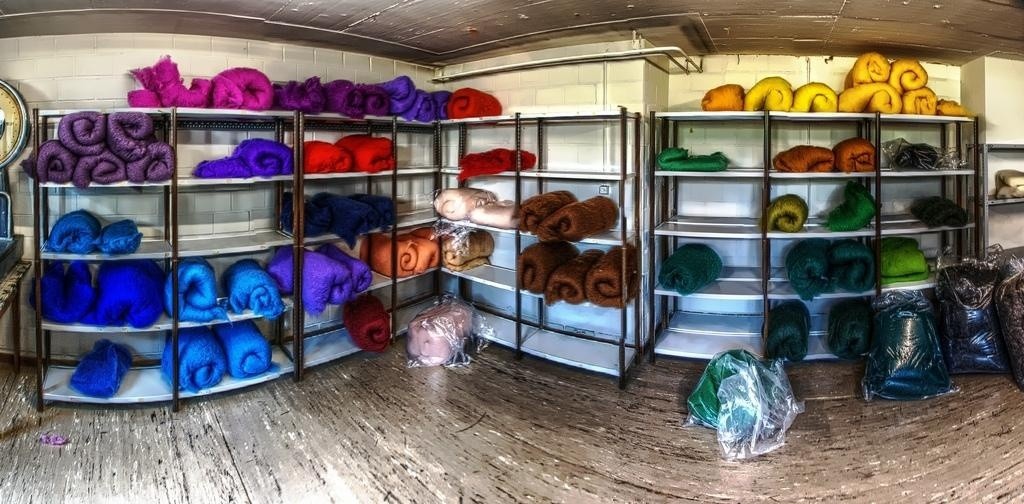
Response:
[0,261,31,374]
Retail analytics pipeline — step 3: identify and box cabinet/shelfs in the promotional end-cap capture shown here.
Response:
[964,142,1023,274]
[298,110,439,378]
[30,103,298,410]
[650,108,979,367]
[437,106,640,389]
